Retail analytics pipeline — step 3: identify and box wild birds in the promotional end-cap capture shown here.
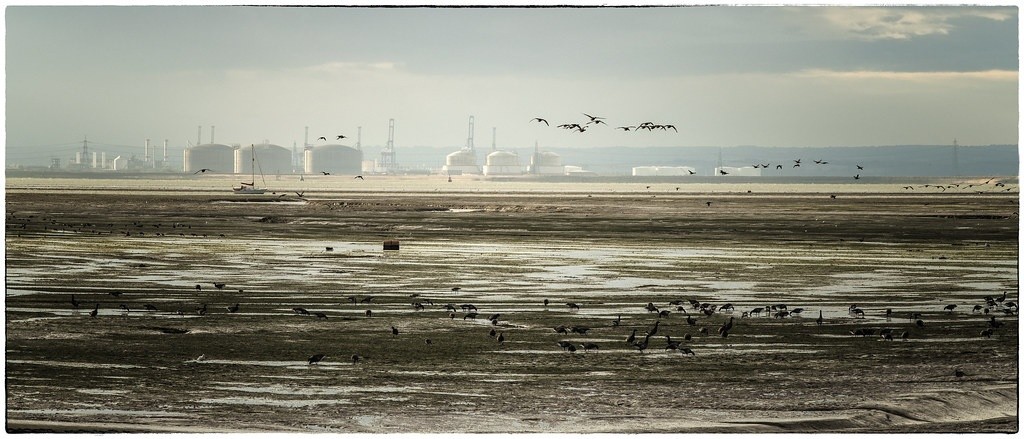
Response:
[542,294,824,352]
[903,176,1020,218]
[194,168,214,174]
[688,157,864,206]
[314,134,364,180]
[308,353,360,367]
[846,290,1018,380]
[73,279,240,321]
[529,111,679,135]
[8,216,226,240]
[292,287,505,346]
